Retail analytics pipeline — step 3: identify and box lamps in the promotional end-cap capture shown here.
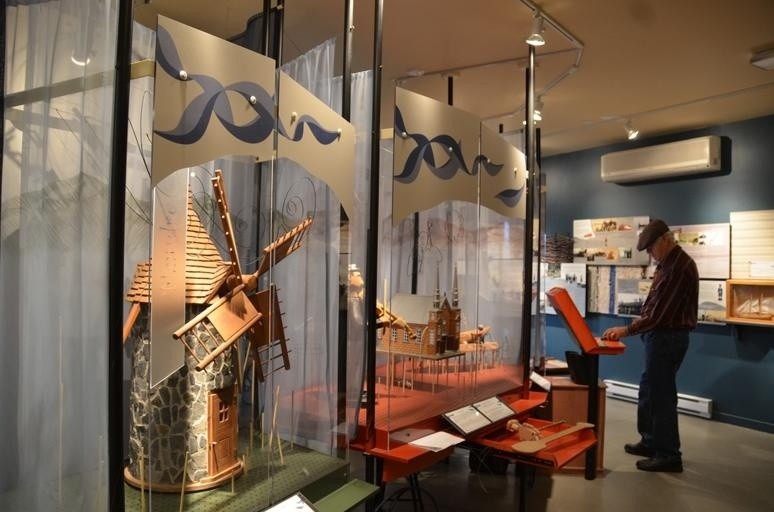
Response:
[533,100,543,120]
[750,49,774,71]
[525,16,547,47]
[624,118,639,140]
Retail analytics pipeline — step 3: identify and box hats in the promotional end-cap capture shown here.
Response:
[636,219,668,251]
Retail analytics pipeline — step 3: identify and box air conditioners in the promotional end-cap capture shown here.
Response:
[601,135,721,182]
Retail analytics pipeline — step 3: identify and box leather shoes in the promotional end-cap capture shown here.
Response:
[637,458,683,471]
[625,444,642,454]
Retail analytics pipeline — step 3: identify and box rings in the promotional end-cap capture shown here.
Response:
[611,332,615,336]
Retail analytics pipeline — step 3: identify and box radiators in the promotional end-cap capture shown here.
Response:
[603,379,713,419]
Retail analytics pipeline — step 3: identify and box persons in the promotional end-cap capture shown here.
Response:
[598,216,698,474]
